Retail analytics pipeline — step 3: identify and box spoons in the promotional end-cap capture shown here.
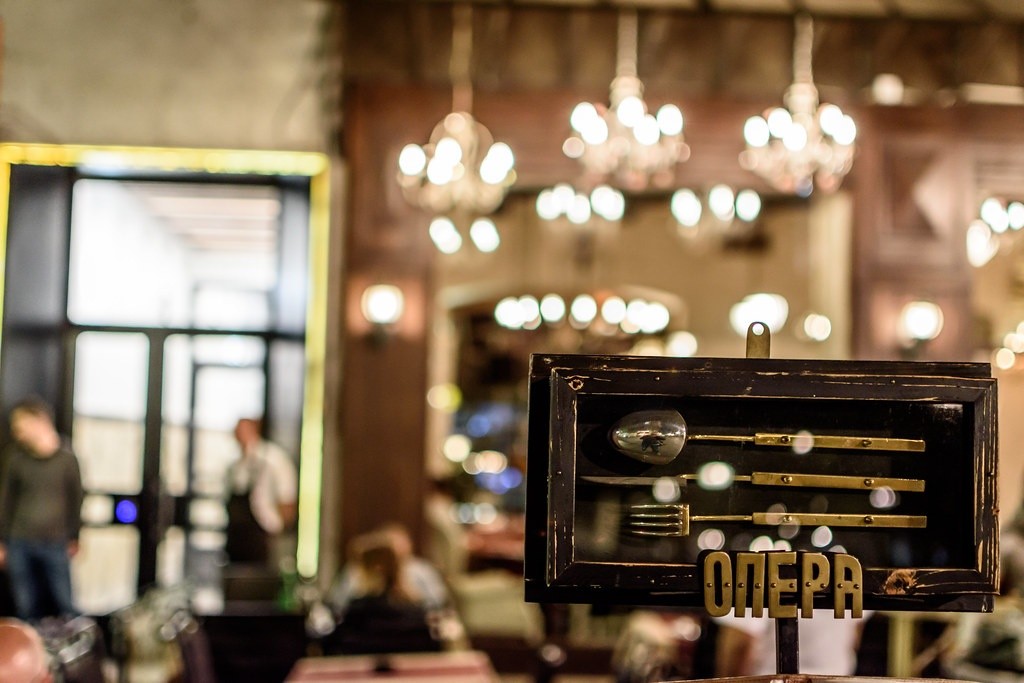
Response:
[609,409,926,465]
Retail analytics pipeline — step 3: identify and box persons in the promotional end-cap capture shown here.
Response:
[0,391,84,624]
[220,417,300,569]
[301,529,879,676]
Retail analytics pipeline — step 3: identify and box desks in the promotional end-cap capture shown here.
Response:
[285,650,504,683]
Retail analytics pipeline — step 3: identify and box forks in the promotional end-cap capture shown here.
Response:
[630,504,927,537]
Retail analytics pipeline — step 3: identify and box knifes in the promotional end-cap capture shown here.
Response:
[580,471,925,493]
